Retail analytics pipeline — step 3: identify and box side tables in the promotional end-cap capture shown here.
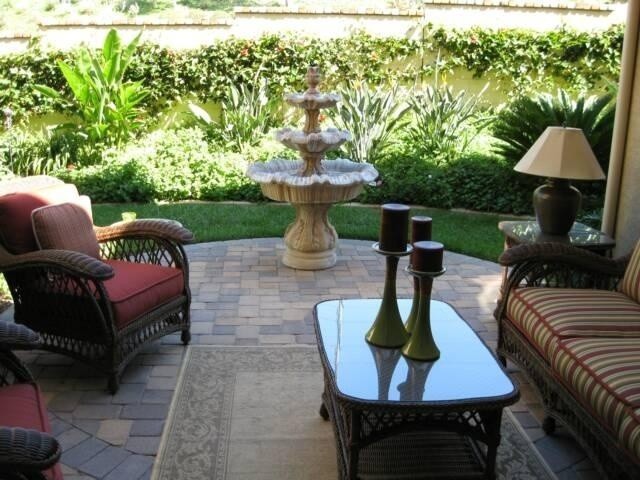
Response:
[498,221,616,293]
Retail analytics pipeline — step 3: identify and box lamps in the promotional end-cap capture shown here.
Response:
[512,122,607,235]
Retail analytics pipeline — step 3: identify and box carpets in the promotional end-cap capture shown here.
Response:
[151,345,560,479]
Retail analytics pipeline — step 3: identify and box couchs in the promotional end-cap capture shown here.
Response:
[493,238,639,480]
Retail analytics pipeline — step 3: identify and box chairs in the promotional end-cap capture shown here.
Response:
[0,320,62,479]
[1,176,194,395]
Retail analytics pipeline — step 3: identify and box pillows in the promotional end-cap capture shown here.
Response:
[31,195,99,281]
